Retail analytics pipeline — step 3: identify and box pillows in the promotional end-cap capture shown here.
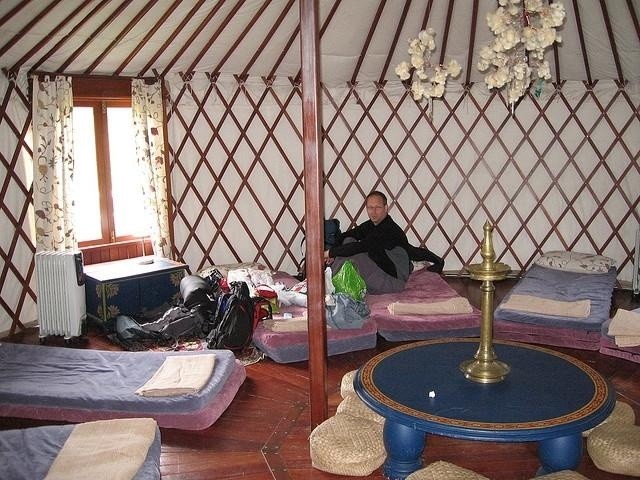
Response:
[197,261,278,281]
[534,249,616,273]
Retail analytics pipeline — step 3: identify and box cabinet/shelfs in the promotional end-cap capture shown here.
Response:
[82,254,189,336]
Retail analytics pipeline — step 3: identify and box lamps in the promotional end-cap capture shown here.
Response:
[395,0,567,117]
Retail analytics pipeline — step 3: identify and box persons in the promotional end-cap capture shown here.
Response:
[323,191,411,294]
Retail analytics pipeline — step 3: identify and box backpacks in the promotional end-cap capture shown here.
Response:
[207,281,272,355]
[298,219,341,283]
